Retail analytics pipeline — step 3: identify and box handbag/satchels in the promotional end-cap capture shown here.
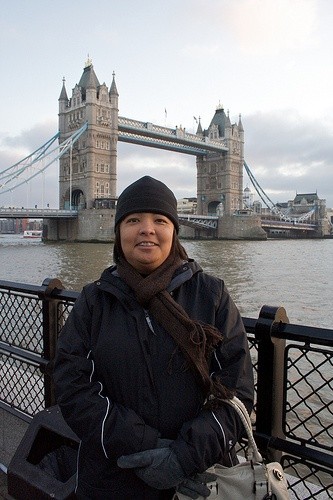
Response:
[172,397,289,500]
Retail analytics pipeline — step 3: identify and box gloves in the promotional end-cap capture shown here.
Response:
[156,439,172,449]
[117,446,184,491]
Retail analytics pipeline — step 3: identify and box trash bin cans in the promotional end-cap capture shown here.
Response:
[9,403,83,500]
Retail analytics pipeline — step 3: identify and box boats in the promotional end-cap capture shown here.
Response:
[23,230,42,238]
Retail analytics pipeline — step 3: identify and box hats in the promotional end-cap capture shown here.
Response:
[114,176,179,232]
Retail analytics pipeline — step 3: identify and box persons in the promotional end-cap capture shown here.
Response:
[51,175,255,500]
